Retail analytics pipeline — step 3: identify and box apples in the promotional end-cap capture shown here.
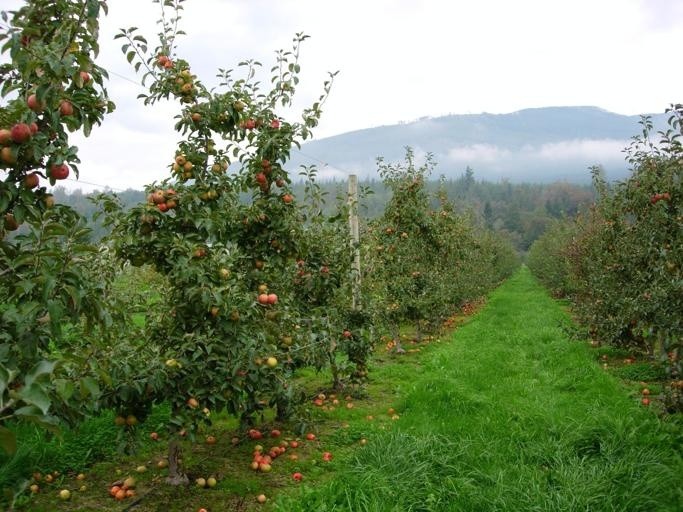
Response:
[23,470,86,500]
[590,192,683,405]
[109,325,400,512]
[0,37,89,231]
[139,56,329,317]
